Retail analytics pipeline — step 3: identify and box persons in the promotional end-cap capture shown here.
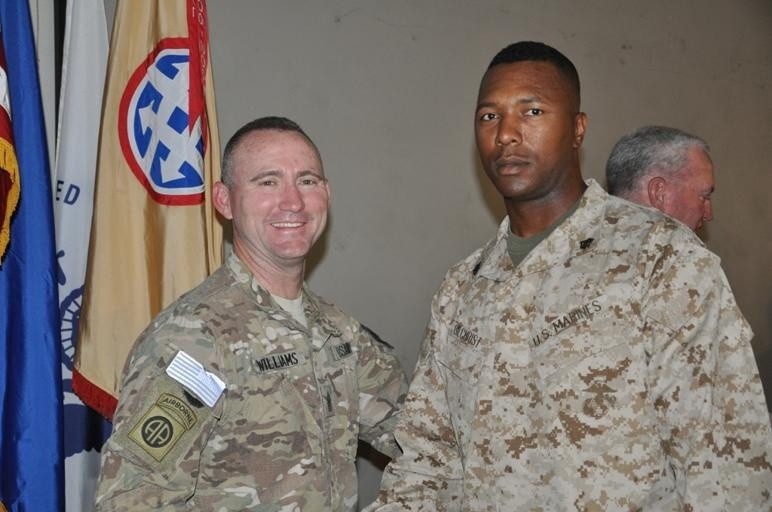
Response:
[93,113,409,509]
[359,37,772,512]
[604,124,717,238]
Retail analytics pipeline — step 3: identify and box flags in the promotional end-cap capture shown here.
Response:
[0,0,222,512]
